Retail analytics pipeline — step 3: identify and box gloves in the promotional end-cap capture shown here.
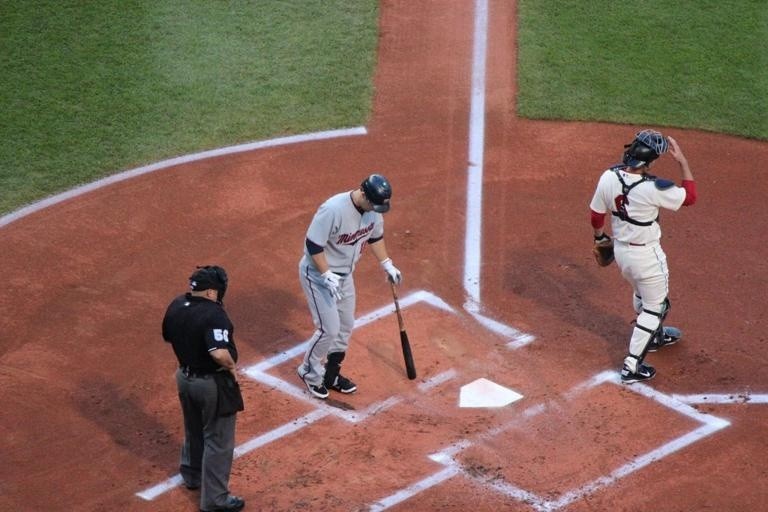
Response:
[321,270,344,300]
[382,259,402,284]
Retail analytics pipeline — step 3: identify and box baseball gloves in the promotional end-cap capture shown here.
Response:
[593,232,614,266]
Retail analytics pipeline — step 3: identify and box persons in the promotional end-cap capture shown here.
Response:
[589,129,697,384]
[162,265,245,512]
[296,174,402,400]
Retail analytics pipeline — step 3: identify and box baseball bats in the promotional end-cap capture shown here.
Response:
[389,275,416,380]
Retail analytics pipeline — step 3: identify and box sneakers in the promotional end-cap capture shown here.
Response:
[649,333,677,352]
[621,365,656,383]
[200,494,244,512]
[325,375,356,394]
[298,373,329,399]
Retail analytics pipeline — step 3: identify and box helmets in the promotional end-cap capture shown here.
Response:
[623,130,669,169]
[362,174,391,212]
[190,265,228,305]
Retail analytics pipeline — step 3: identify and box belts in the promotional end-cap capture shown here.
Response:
[183,370,205,378]
